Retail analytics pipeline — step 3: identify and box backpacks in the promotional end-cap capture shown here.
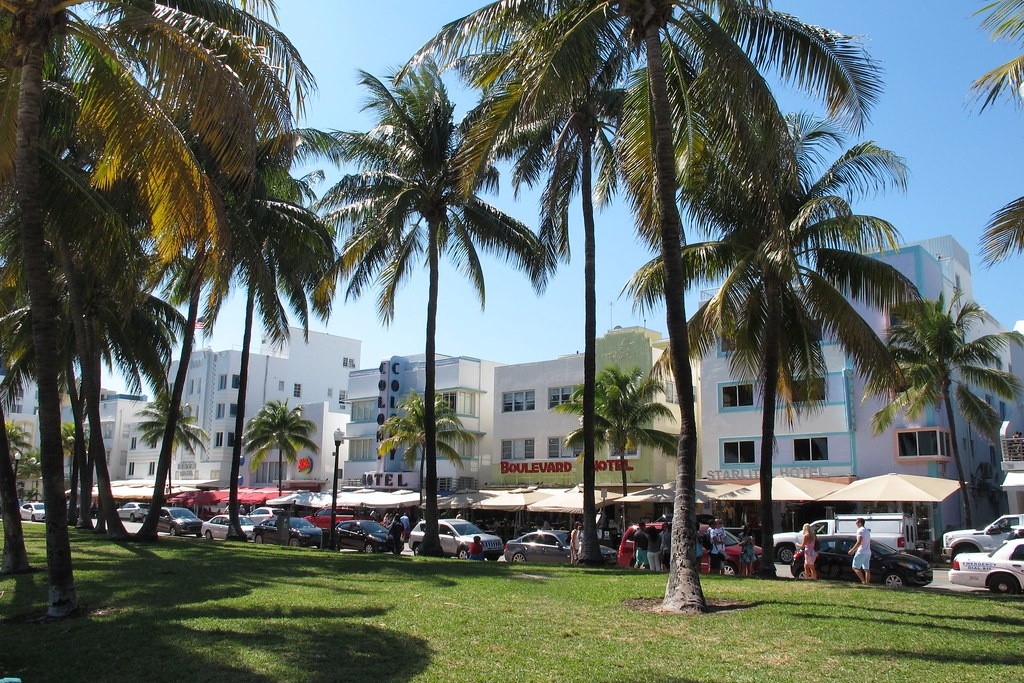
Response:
[701,530,711,549]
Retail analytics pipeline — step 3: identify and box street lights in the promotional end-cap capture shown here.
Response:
[14,451,21,481]
[329,428,343,550]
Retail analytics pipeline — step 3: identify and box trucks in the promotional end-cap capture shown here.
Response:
[941,513,1024,565]
[773,513,916,565]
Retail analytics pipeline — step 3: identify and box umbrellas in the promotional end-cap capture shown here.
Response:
[817,473,969,513]
[613,480,744,504]
[526,485,622,530]
[166,491,286,514]
[717,476,847,531]
[65,484,201,499]
[419,488,555,530]
[266,490,441,515]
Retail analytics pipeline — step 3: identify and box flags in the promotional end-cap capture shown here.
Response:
[195,316,205,328]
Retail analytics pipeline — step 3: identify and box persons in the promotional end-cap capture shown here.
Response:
[292,500,297,511]
[469,536,484,560]
[489,520,501,528]
[215,510,222,515]
[633,501,757,576]
[848,518,871,583]
[795,523,816,580]
[1008,432,1022,454]
[569,521,582,564]
[456,511,463,519]
[199,508,209,521]
[366,511,410,554]
[239,505,245,515]
[542,517,551,530]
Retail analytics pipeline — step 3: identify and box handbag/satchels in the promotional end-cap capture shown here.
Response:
[717,551,726,561]
[813,539,820,552]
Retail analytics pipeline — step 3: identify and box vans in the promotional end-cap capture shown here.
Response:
[617,523,764,577]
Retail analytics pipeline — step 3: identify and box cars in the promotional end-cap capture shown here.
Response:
[504,530,617,568]
[790,536,933,590]
[200,515,255,544]
[252,517,322,547]
[246,506,285,524]
[19,503,46,522]
[143,506,203,536]
[117,502,151,522]
[334,520,405,554]
[947,526,1024,594]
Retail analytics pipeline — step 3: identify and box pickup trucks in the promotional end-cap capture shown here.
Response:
[303,505,375,532]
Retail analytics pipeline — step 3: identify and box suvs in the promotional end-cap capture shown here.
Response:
[407,519,505,561]
